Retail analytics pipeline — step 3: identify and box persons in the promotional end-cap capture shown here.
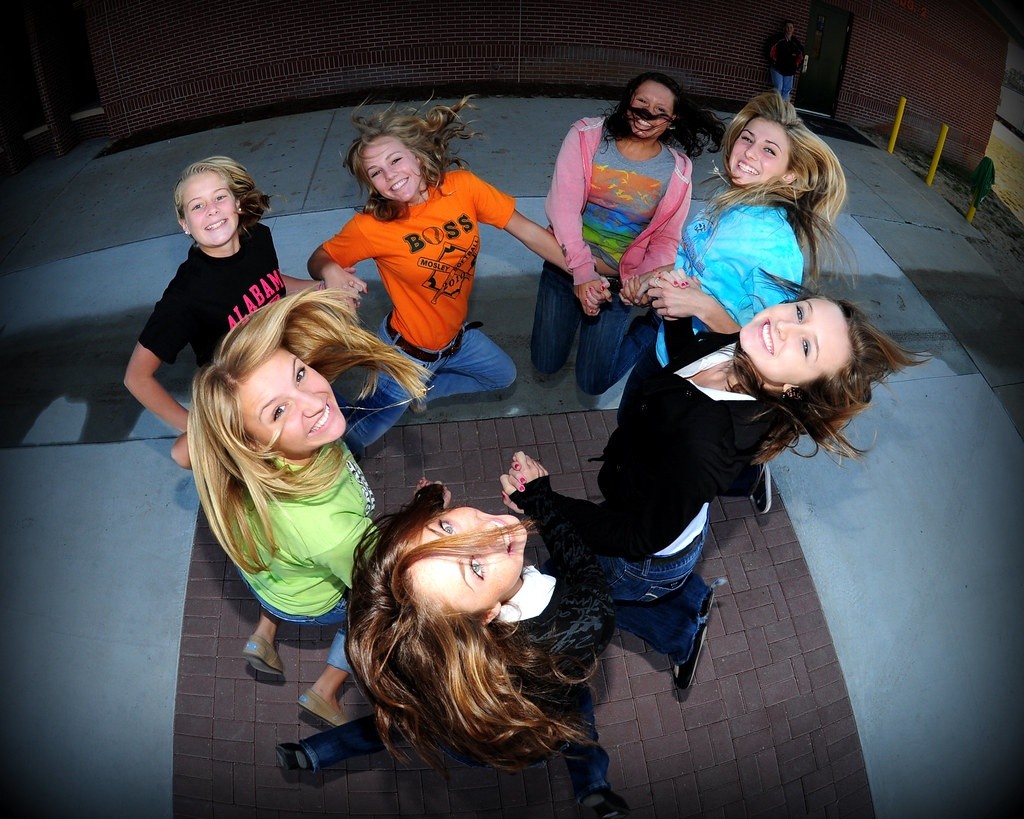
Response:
[274,450,631,819]
[307,91,613,456]
[530,71,736,396]
[500,267,933,692]
[616,90,860,514]
[170,285,432,729]
[124,155,368,432]
[762,20,806,104]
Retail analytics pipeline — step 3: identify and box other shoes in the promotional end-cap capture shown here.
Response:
[409,400,428,416]
[750,464,772,514]
[243,633,283,675]
[578,785,630,819]
[296,687,352,729]
[275,743,313,783]
[673,622,707,690]
[699,588,715,617]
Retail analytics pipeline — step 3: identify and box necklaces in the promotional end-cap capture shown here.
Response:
[338,385,436,447]
[710,160,732,191]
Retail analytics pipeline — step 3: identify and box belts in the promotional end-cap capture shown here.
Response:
[386,310,485,363]
[650,528,702,565]
[604,276,623,295]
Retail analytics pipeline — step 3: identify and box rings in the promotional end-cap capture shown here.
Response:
[654,276,662,287]
